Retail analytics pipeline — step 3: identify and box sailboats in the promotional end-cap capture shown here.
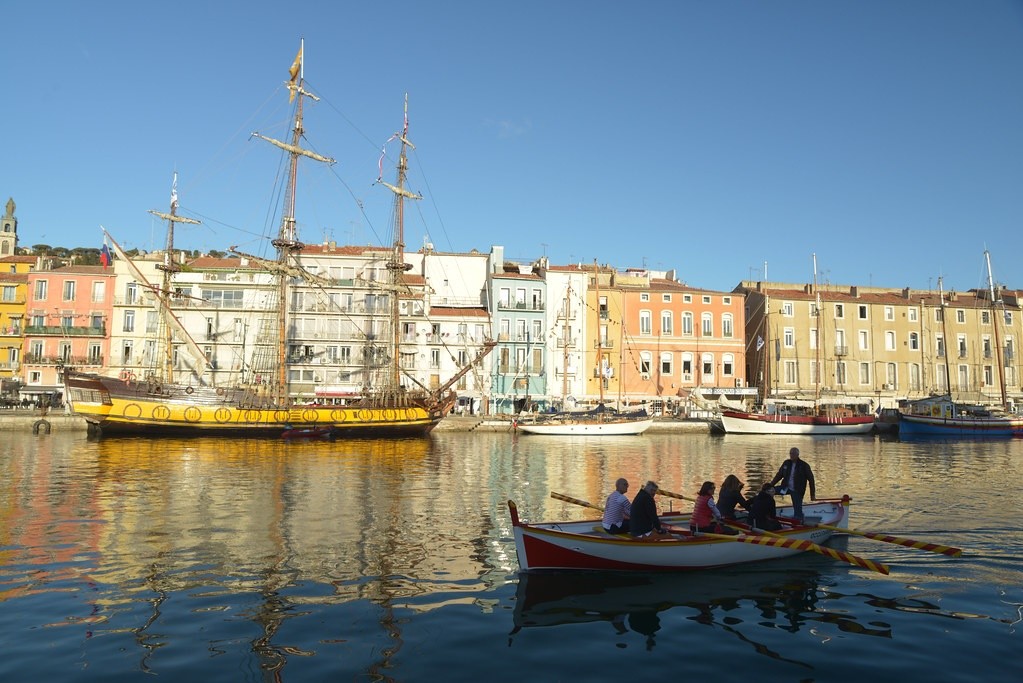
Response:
[64,37,498,440]
[511,257,657,435]
[718,250,1023,435]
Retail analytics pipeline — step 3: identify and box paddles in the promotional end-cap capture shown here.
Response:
[668,529,815,551]
[551,492,605,512]
[778,519,963,557]
[640,484,695,502]
[721,520,890,575]
[33,420,50,431]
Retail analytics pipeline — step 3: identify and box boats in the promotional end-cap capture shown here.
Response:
[508,499,849,574]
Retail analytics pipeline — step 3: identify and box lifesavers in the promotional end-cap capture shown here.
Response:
[127,374,137,380]
[216,387,224,395]
[118,371,129,380]
[185,387,194,394]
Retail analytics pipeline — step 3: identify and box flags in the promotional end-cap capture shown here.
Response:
[100,235,112,271]
[170,174,177,207]
[289,46,301,102]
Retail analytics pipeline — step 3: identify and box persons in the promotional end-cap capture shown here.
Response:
[771,447,815,527]
[716,475,751,524]
[690,481,738,535]
[602,478,632,534]
[746,484,783,531]
[630,481,671,538]
